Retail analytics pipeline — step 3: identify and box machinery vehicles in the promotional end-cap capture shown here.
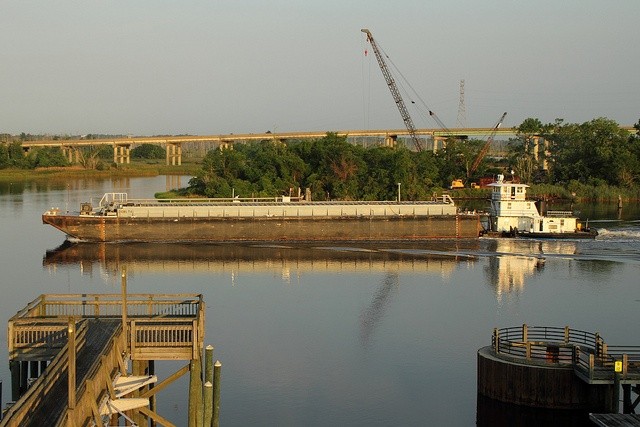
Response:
[449,112,508,189]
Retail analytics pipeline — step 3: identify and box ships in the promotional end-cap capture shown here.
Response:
[41,169,599,245]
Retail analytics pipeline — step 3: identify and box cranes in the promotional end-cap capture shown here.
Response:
[360,28,451,156]
